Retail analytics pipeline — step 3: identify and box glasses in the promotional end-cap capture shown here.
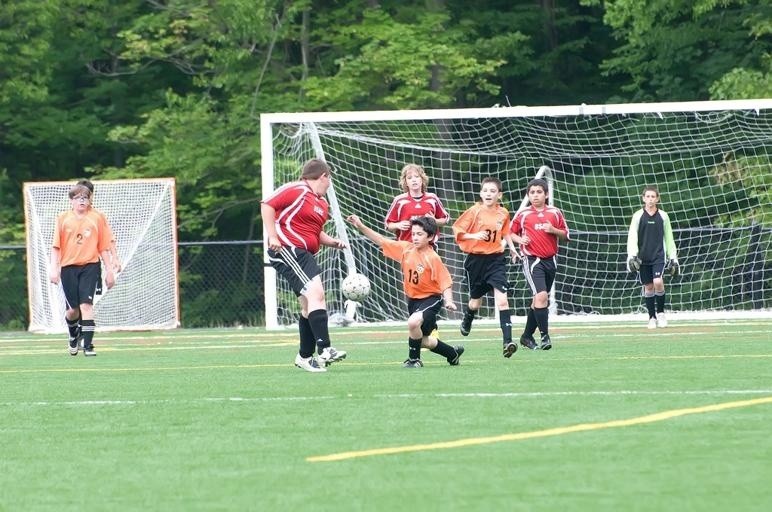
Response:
[72,196,89,200]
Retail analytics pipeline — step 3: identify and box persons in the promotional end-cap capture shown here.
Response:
[383,163,451,338]
[49,184,115,356]
[346,213,465,367]
[510,178,570,350]
[259,158,347,373]
[627,187,679,330]
[452,177,523,358]
[77,179,122,350]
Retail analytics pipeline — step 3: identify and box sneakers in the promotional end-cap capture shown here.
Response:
[319,347,346,367]
[541,335,551,351]
[403,358,424,369]
[294,353,327,372]
[520,335,539,350]
[503,342,518,357]
[84,344,96,356]
[69,337,79,355]
[447,346,464,365]
[461,318,473,336]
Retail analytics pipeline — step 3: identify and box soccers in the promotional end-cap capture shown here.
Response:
[341,273,371,302]
